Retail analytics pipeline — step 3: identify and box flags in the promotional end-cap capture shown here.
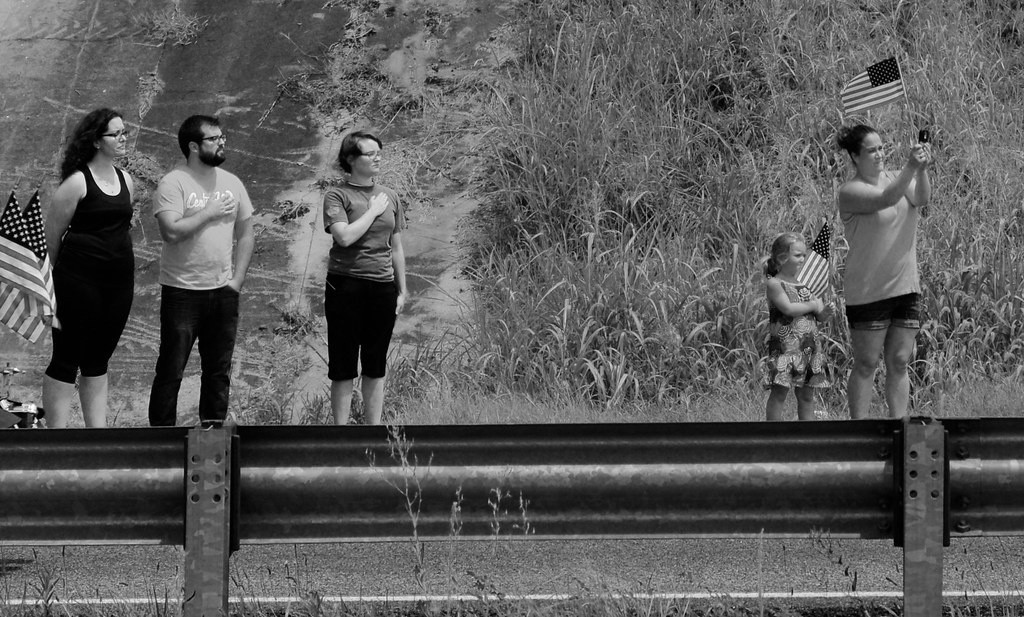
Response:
[841,59,905,114]
[0,192,53,343]
[798,223,830,298]
[23,191,60,327]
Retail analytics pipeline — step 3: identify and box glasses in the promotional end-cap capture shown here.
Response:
[202,135,226,143]
[103,129,128,139]
[362,151,382,159]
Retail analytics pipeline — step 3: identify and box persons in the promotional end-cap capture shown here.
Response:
[836,125,932,419]
[764,231,830,420]
[323,131,408,425]
[149,115,255,427]
[44,108,133,428]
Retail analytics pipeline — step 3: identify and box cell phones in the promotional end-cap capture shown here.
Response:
[919,130,929,143]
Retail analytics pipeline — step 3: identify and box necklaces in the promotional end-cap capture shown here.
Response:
[105,183,108,186]
